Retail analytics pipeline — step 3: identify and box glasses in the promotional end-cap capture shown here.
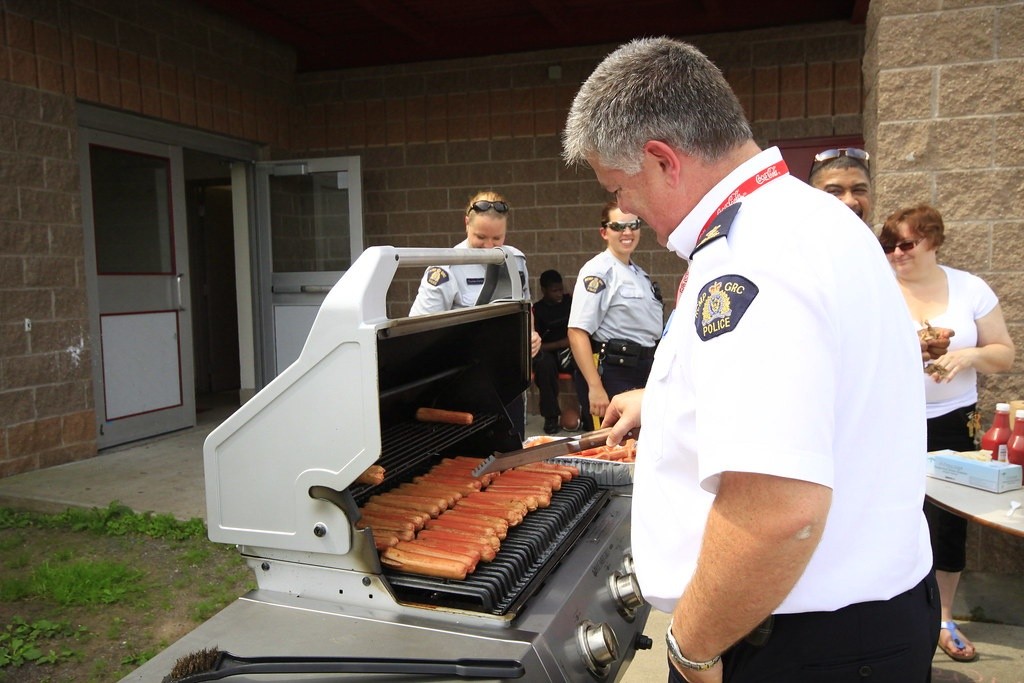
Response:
[467,200,508,216]
[807,148,871,181]
[604,220,642,231]
[884,236,924,253]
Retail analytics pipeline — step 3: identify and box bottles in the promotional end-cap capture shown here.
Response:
[979,401,1014,464]
[1006,410,1024,488]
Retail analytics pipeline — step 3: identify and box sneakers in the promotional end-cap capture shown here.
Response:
[544,416,560,434]
[581,412,594,431]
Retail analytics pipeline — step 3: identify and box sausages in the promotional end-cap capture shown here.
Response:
[360,434,637,580]
[416,408,473,426]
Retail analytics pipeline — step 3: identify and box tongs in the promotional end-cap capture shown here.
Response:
[471,416,637,478]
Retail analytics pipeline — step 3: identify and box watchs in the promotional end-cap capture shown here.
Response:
[665,620,720,670]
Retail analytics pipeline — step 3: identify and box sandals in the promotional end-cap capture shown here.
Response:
[939,621,976,660]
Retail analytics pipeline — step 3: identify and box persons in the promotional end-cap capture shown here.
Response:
[405,189,542,360]
[568,200,666,434]
[559,37,942,682]
[531,271,594,437]
[809,146,871,225]
[880,205,1018,662]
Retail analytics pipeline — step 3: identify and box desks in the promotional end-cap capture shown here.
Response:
[925,450,1024,538]
